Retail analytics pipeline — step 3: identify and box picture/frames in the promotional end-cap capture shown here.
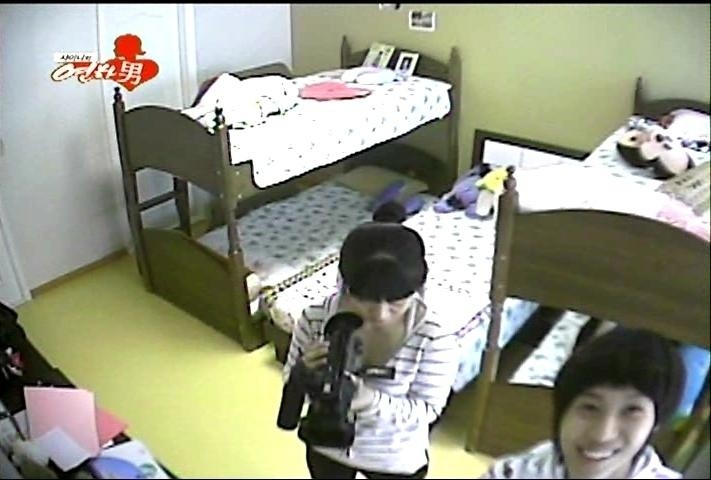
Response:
[408,10,435,32]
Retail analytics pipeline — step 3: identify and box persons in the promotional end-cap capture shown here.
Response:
[276,199,464,480]
[369,160,518,223]
[477,326,688,478]
[399,56,412,70]
[370,49,382,67]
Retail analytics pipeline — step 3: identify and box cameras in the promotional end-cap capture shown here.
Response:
[276,311,363,444]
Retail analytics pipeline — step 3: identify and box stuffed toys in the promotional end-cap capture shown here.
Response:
[611,104,709,182]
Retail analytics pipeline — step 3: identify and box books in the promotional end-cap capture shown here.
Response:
[84,438,168,480]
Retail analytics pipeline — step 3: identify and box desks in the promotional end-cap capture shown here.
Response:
[0,298,180,478]
